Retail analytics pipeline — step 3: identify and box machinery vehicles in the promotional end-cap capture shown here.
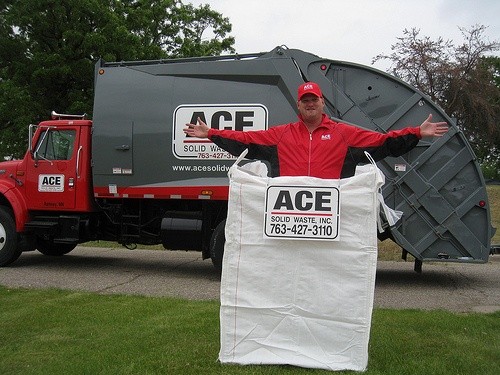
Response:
[0,43,498,274]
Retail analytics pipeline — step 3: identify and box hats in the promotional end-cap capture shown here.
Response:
[298,81,322,102]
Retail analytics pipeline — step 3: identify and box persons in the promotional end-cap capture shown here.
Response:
[183,82,449,180]
